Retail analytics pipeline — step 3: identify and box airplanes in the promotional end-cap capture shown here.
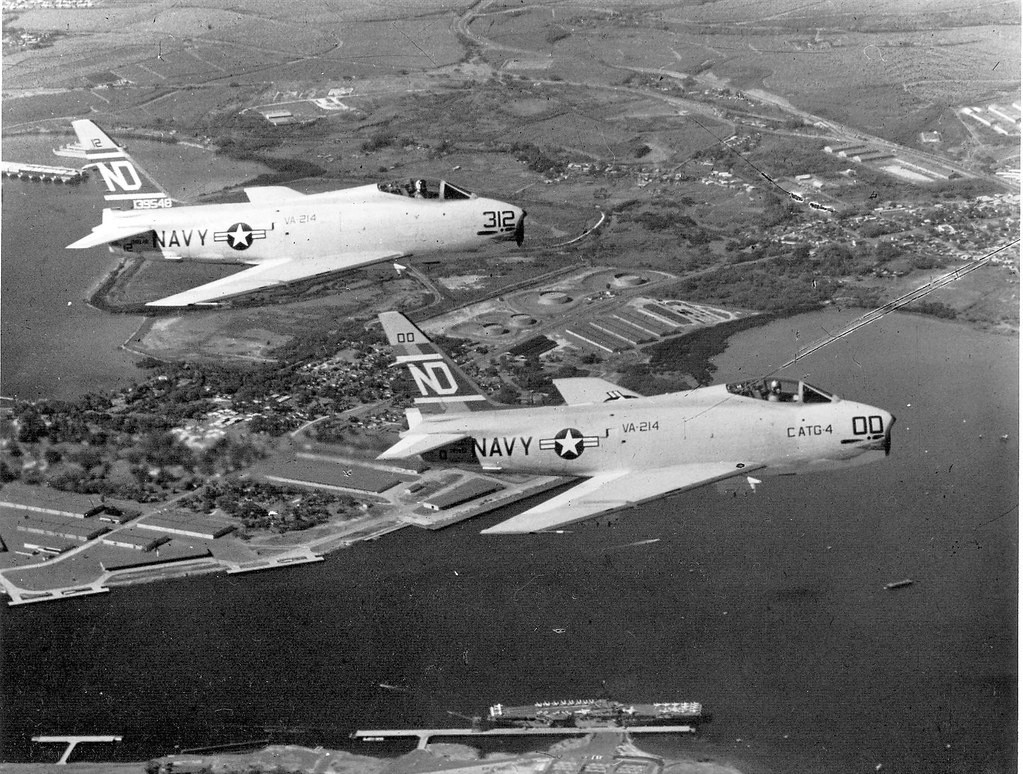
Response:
[65,117,529,309]
[370,307,900,535]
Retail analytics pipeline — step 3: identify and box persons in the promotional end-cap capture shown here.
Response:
[767,380,787,403]
[414,178,432,199]
